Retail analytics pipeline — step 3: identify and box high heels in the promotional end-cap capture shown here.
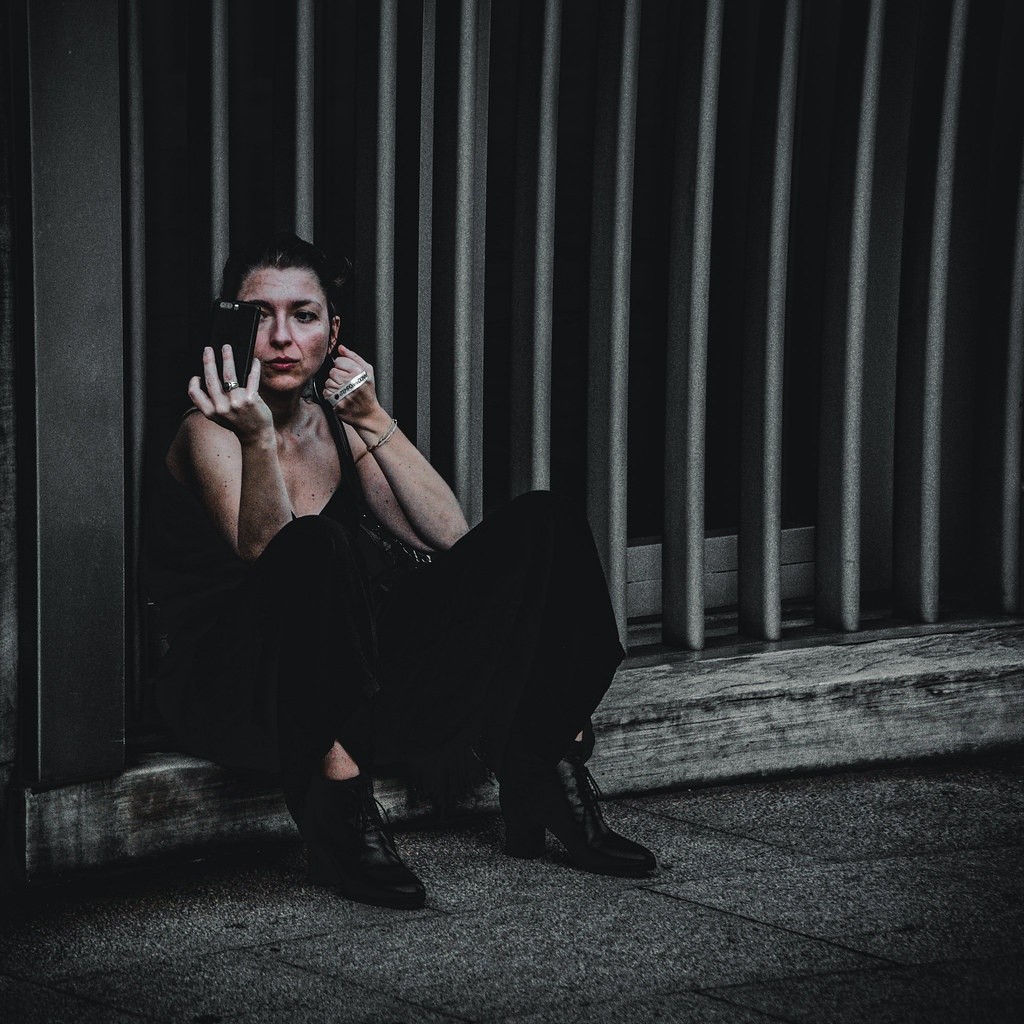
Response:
[298,768,426,904]
[499,745,656,871]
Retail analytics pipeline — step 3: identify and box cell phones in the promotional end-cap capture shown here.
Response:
[198,299,260,397]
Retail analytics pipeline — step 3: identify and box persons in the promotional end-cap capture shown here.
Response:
[137,232,657,907]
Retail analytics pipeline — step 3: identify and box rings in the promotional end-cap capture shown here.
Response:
[224,382,239,391]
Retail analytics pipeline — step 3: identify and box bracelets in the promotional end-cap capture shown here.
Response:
[367,419,397,451]
